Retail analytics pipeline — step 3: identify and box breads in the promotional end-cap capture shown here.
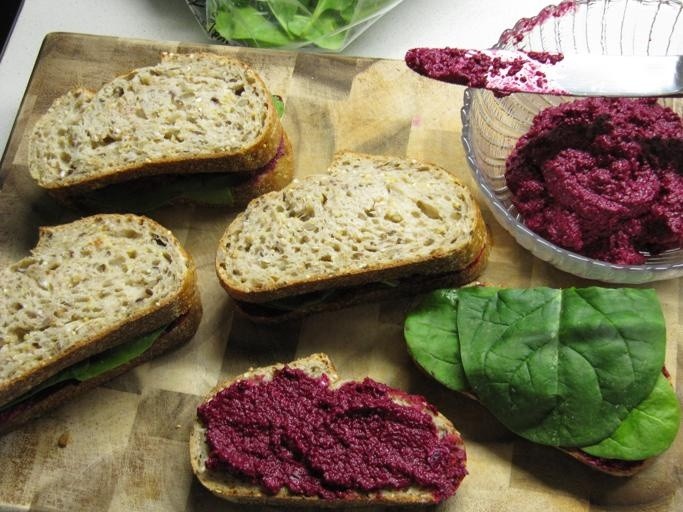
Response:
[0,213,202,436]
[216,151,492,324]
[188,352,466,506]
[28,52,295,213]
[408,279,676,480]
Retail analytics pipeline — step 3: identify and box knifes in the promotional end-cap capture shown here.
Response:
[402,47,683,98]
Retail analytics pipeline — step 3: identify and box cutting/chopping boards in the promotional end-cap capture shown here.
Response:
[0,31,683,512]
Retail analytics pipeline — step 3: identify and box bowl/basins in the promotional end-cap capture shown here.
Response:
[460,0,683,286]
